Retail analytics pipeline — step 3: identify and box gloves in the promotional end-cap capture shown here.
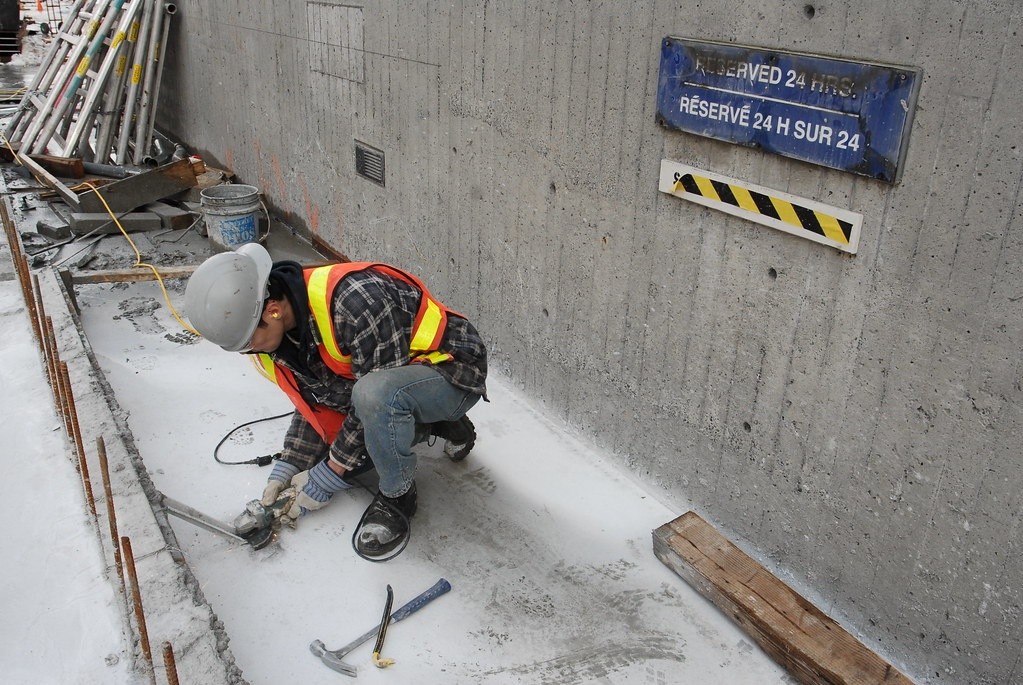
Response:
[287,456,353,519]
[262,461,300,530]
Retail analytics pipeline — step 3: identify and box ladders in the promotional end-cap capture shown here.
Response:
[2,0,166,167]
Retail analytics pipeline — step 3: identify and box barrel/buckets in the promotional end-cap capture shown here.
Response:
[21,36,44,66]
[201,184,261,255]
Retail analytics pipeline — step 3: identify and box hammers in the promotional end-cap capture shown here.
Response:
[309,576,450,678]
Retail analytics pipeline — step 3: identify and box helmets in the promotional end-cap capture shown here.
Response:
[184,243,273,352]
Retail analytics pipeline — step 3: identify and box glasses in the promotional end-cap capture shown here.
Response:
[238,337,255,353]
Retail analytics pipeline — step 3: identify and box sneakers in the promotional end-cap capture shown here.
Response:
[427,415,476,463]
[358,482,417,555]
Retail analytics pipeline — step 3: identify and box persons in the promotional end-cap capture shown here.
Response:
[185,252,487,555]
